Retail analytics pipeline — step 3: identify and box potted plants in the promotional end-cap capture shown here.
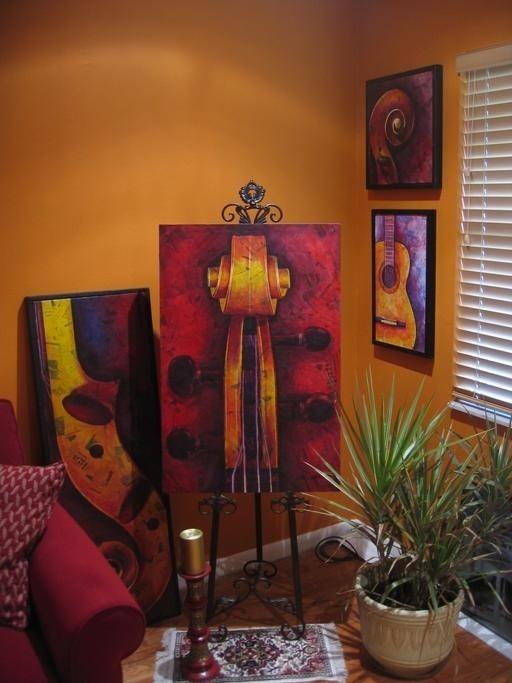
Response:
[292,363,512,678]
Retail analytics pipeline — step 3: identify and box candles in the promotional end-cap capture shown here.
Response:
[179,528,206,576]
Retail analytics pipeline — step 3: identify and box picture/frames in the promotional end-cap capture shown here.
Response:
[25,287,182,625]
[371,209,436,359]
[365,64,442,190]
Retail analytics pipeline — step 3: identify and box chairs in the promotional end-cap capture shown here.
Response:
[0,399,146,683]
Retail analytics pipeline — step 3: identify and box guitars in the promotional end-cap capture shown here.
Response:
[375,214,416,351]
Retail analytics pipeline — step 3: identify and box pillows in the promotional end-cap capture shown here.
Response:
[0,459,69,632]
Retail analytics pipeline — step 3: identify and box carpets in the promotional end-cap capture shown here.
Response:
[152,622,349,683]
